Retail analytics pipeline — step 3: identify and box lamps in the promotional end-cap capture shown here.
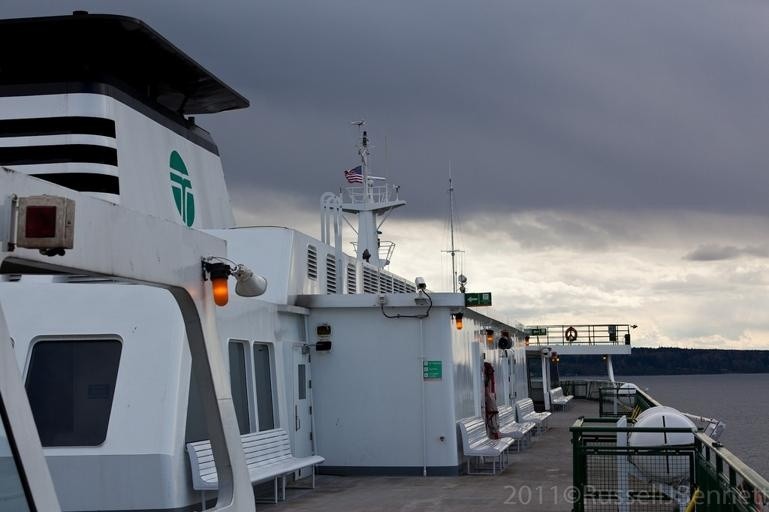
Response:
[199,256,268,307]
[451,312,463,330]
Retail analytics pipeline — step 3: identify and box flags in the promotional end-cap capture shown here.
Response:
[343,166,364,184]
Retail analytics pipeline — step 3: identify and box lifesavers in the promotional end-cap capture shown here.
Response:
[566,328,577,341]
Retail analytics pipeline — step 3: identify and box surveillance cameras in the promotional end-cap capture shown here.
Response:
[415,277,426,289]
[395,186,400,188]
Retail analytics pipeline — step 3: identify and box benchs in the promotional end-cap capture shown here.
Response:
[548,385,574,414]
[186,427,326,511]
[458,397,553,476]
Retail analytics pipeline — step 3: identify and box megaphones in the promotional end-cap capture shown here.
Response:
[230,264,267,297]
